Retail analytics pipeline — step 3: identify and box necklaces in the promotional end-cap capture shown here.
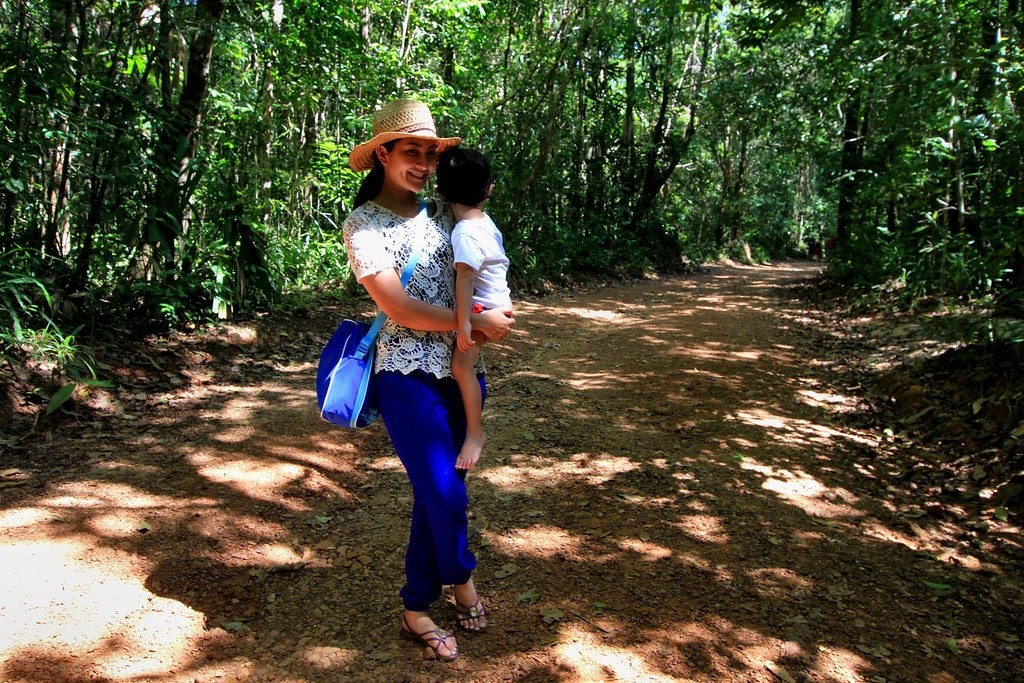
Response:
[378,197,419,216]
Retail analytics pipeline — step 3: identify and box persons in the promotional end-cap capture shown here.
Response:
[342,99,517,661]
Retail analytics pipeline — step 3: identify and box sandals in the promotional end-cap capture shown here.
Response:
[454,593,488,633]
[399,605,457,661]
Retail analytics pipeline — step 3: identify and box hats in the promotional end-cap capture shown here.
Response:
[349,100,460,171]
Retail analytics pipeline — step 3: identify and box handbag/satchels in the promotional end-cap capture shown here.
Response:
[317,318,376,430]
